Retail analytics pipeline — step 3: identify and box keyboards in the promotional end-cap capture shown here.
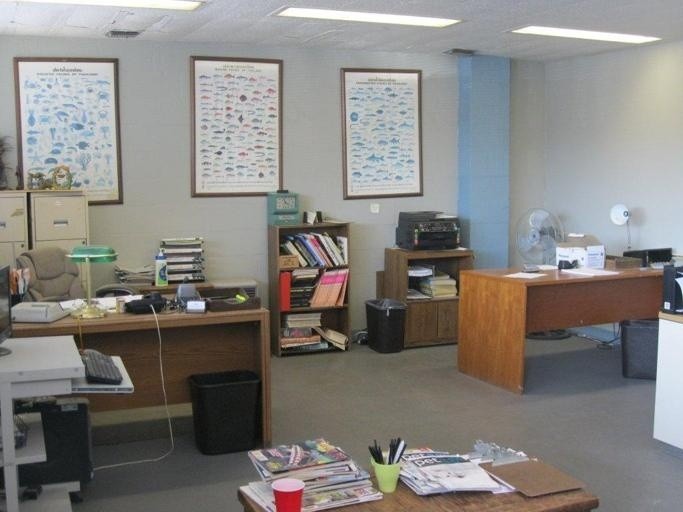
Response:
[83,350,123,384]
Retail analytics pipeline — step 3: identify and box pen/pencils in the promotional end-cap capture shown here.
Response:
[368,440,384,464]
[388,437,407,463]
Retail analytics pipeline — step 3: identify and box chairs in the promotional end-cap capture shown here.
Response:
[15,246,88,301]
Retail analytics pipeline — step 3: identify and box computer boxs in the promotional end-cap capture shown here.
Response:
[0,396,95,492]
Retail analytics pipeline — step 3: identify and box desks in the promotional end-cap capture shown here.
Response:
[457,259,668,395]
[10,294,270,448]
[0,335,134,512]
[238,451,600,511]
[652,310,683,448]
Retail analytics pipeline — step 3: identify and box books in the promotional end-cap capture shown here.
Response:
[280,231,349,268]
[381,447,500,495]
[278,268,350,311]
[247,437,383,512]
[279,313,347,351]
[407,264,432,276]
[420,270,458,298]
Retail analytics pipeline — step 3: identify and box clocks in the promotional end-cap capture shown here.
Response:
[268,191,299,223]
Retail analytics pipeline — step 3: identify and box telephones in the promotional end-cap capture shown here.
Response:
[125,290,167,314]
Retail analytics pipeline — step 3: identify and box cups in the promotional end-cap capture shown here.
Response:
[369,457,401,493]
[271,478,305,512]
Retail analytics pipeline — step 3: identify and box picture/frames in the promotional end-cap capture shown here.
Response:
[189,54,284,198]
[340,68,423,200]
[13,56,124,206]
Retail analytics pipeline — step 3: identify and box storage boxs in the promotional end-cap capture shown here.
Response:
[0,190,92,299]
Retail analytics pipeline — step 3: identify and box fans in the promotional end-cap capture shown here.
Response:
[514,207,571,341]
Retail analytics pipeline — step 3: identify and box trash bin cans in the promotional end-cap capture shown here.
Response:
[364,299,408,353]
[620,319,659,379]
[188,369,260,455]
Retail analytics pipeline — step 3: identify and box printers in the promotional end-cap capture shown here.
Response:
[394,210,462,251]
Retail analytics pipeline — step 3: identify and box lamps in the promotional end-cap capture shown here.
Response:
[610,204,636,250]
[1,1,203,12]
[276,6,461,30]
[511,24,662,45]
[65,246,120,320]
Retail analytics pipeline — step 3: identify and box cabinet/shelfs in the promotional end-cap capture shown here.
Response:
[376,212,475,350]
[269,224,350,357]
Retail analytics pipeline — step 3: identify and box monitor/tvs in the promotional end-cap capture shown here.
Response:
[0,264,13,357]
